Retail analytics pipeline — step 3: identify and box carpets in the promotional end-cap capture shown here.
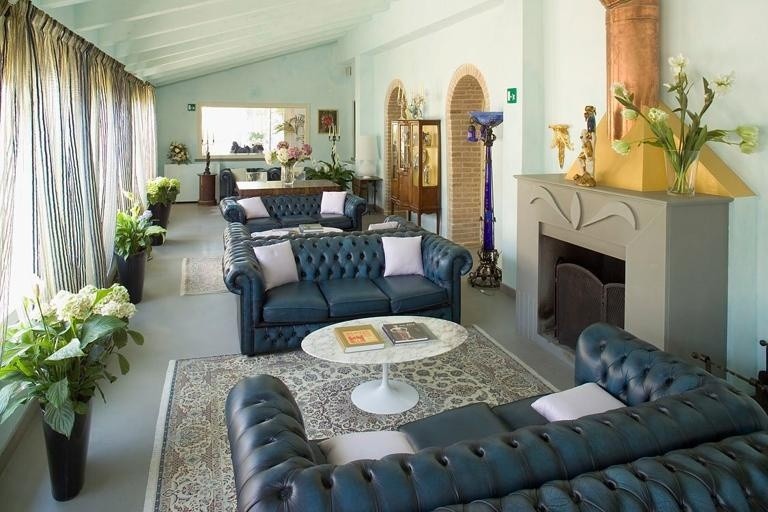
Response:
[180,253,230,297]
[140,323,558,512]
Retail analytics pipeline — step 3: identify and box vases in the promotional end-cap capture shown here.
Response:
[149,202,171,228]
[281,166,295,185]
[664,150,702,195]
[38,405,94,503]
[114,250,148,304]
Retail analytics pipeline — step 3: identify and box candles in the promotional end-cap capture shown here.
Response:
[338,127,340,136]
[333,125,336,136]
[329,125,331,136]
[398,80,400,98]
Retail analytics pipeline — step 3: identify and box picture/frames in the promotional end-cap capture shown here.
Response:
[317,108,342,137]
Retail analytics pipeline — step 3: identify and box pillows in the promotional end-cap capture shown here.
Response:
[250,231,288,236]
[317,431,415,463]
[236,196,271,218]
[250,240,300,291]
[230,168,247,181]
[368,221,398,232]
[530,380,629,423]
[319,191,347,215]
[381,236,424,276]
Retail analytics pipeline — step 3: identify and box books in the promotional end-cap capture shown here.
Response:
[334,324,384,353]
[299,222,324,234]
[382,321,429,346]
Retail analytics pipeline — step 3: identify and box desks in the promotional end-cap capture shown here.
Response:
[197,172,217,206]
[235,179,341,196]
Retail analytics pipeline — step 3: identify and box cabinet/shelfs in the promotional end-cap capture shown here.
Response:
[352,176,383,213]
[164,162,220,204]
[389,120,440,235]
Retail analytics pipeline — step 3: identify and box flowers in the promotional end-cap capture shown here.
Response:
[0,276,144,439]
[146,175,179,205]
[608,49,760,191]
[114,193,167,263]
[265,141,312,167]
[165,139,191,165]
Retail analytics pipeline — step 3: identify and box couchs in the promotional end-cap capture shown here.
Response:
[224,321,767,511]
[221,193,367,230]
[222,167,281,195]
[435,429,762,512]
[223,229,472,356]
[221,213,420,243]
